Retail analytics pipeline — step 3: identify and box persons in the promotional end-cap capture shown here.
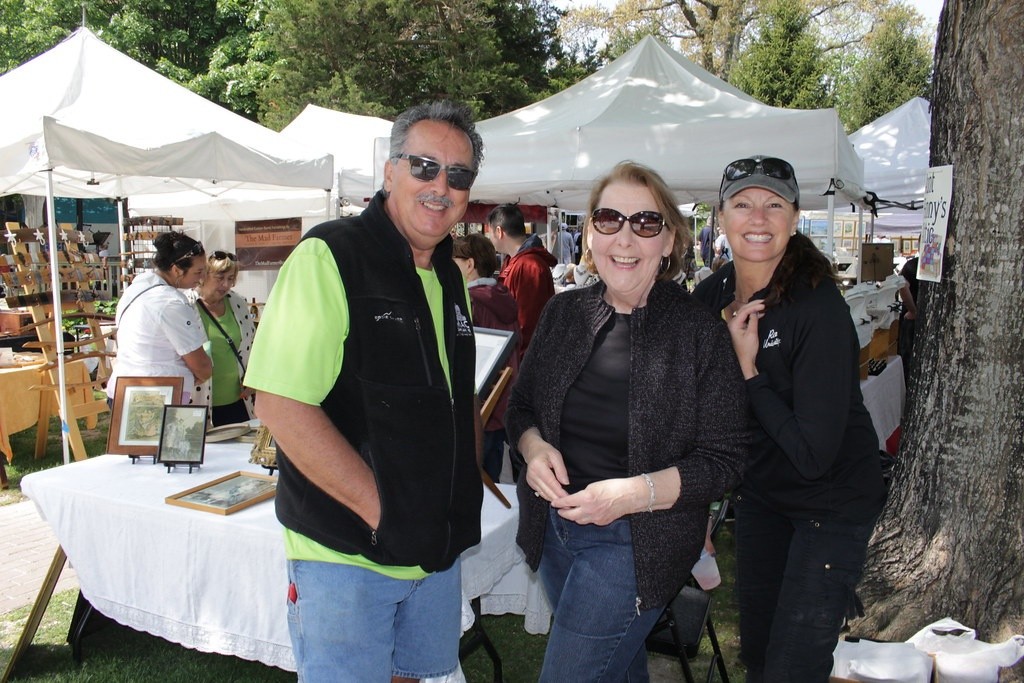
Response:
[183,253,257,427]
[507,164,748,683]
[690,156,888,682]
[699,215,731,272]
[449,201,583,481]
[106,232,212,407]
[243,101,483,683]
[897,234,921,383]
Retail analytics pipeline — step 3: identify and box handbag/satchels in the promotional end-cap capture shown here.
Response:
[905,616,1024,683]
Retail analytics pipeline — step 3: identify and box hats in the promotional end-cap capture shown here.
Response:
[561,223,568,229]
[719,154,800,207]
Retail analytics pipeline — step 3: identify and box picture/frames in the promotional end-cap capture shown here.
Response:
[166,469,277,516]
[158,403,209,465]
[250,421,280,465]
[108,376,182,456]
[472,324,515,395]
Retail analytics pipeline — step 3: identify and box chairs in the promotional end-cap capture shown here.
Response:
[643,498,732,683]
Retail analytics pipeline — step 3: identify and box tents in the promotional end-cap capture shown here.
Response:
[373,34,863,284]
[800,95,930,242]
[124,101,392,222]
[559,204,698,262]
[0,31,333,465]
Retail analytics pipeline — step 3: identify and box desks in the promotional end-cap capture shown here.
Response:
[5,445,553,683]
[859,355,905,457]
[0,350,96,488]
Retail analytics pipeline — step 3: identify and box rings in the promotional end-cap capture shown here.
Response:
[535,491,542,497]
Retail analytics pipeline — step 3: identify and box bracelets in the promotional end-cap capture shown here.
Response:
[642,473,655,513]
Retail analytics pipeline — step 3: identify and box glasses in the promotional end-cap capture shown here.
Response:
[170,241,203,267]
[590,208,668,238]
[718,158,799,200]
[207,251,237,263]
[391,153,477,190]
